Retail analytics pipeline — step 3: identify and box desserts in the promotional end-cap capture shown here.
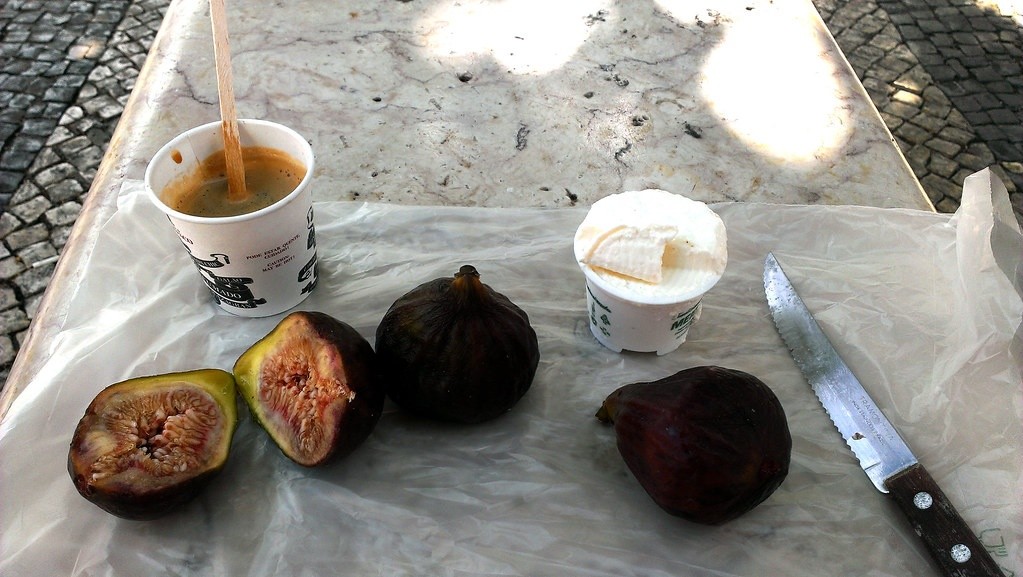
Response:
[575,188,728,302]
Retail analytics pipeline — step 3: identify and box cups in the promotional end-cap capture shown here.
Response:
[573,200,727,357]
[145,118,320,318]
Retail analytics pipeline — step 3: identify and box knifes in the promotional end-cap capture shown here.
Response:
[764,251,1009,577]
